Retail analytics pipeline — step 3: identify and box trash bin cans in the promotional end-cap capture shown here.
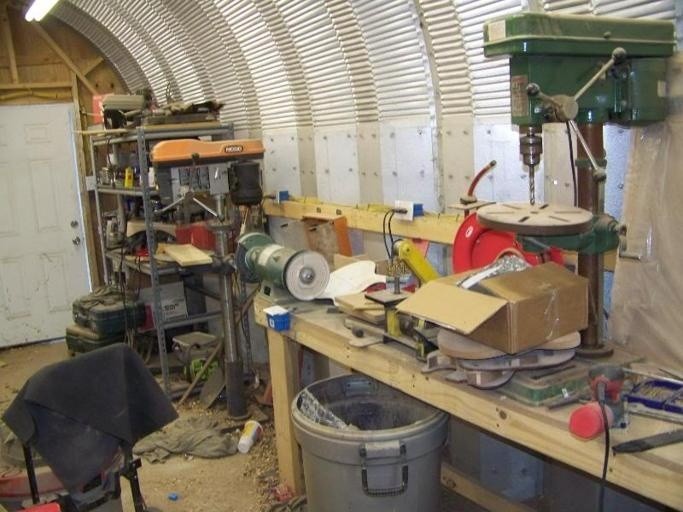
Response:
[290,372,450,512]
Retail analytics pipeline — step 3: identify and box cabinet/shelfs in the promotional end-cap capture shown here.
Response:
[89,121,255,402]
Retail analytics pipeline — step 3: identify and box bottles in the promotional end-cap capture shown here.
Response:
[129,149,140,179]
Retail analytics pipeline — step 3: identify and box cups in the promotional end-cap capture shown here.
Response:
[237,420,264,453]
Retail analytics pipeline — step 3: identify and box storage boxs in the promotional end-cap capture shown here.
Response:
[393,257,591,356]
[171,329,218,365]
[133,280,192,332]
[101,92,145,129]
[150,139,266,205]
[63,289,147,354]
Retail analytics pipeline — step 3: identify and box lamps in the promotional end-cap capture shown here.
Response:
[21,0,63,24]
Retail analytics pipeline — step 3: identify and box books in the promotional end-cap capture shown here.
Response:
[163,243,213,267]
[335,291,407,325]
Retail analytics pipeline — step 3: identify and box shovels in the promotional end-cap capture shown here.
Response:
[199,366,225,410]
